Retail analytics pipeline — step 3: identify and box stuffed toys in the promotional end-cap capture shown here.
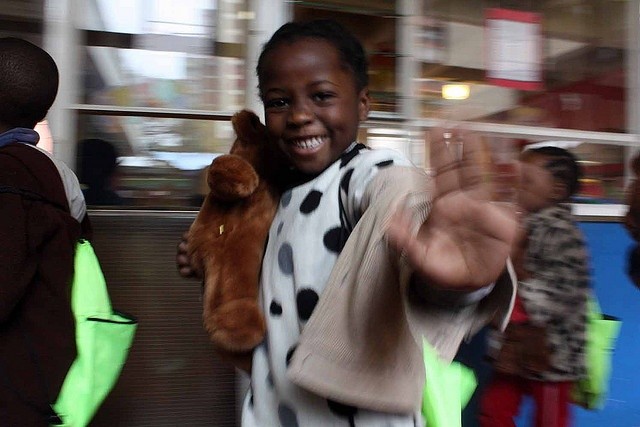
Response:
[184,108,285,354]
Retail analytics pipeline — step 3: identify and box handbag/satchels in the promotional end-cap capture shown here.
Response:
[422,339,478,426]
[568,291,621,411]
[51,238,139,426]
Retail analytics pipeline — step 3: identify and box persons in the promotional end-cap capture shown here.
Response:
[77,139,124,205]
[6,27,93,425]
[177,21,525,426]
[475,146,590,427]
